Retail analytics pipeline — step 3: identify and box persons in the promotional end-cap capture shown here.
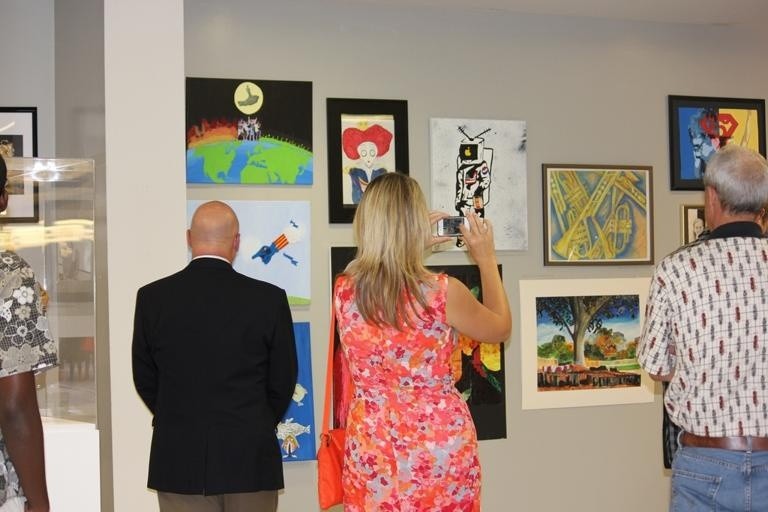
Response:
[635,144,768,512]
[0,155,61,512]
[132,200,298,512]
[333,173,512,512]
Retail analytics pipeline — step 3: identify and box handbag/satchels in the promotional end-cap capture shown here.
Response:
[320,429,345,511]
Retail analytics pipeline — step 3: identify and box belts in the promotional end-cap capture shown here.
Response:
[679,432,766,452]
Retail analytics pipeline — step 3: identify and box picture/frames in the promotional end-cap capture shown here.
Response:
[679,204,707,247]
[542,163,654,266]
[667,94,766,191]
[0,106,39,223]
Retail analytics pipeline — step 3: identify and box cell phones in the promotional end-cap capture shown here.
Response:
[437,216,471,237]
